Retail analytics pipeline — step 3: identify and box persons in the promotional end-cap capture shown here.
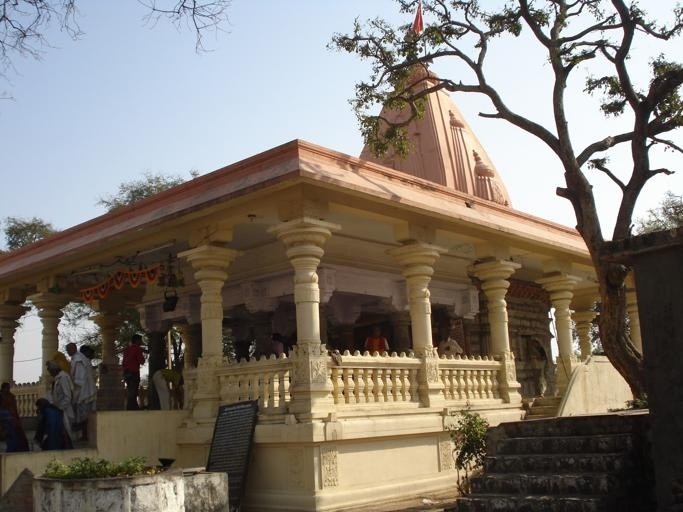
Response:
[362,323,389,355]
[0,334,185,452]
[437,330,462,357]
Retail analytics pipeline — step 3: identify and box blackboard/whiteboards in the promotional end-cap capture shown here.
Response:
[206,400,259,506]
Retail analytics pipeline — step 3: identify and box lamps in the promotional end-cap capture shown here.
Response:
[156,456,177,470]
[161,287,180,312]
